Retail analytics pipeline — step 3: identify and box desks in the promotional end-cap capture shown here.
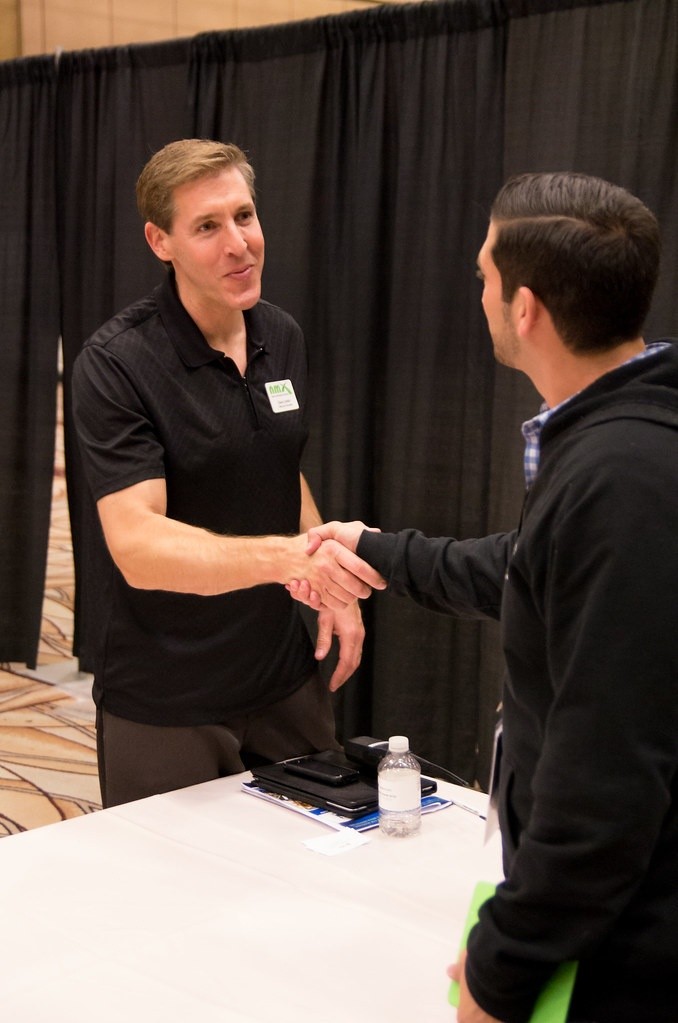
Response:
[0,769,506,1023]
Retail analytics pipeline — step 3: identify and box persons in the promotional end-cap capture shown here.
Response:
[67,140,678,1023]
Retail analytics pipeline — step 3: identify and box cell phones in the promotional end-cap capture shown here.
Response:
[282,757,361,786]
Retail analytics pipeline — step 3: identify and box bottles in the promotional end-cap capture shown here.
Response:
[377,736,421,836]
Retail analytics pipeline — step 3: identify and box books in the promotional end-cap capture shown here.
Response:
[241,782,453,833]
